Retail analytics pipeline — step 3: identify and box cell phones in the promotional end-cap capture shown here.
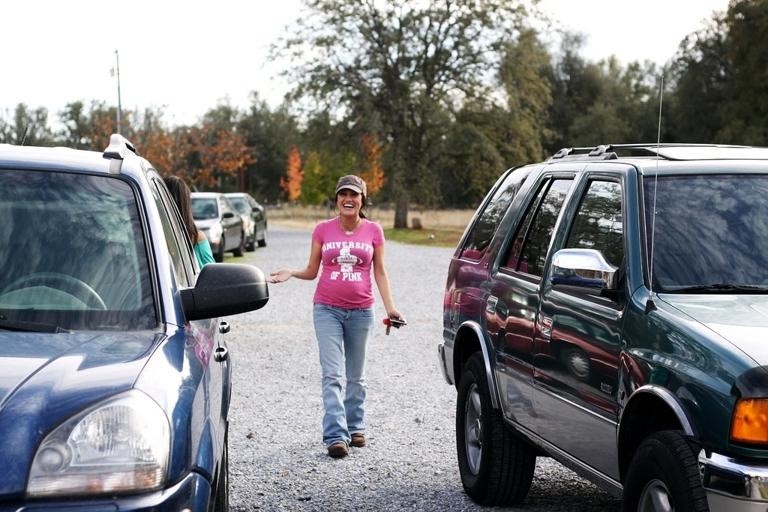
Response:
[388,317,407,325]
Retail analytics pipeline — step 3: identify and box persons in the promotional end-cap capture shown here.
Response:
[266,174,405,455]
[183,321,213,423]
[163,175,216,269]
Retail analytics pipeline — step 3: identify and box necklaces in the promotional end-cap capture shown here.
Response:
[340,218,360,235]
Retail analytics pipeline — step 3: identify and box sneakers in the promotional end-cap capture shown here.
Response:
[351,432,366,446]
[327,441,349,458]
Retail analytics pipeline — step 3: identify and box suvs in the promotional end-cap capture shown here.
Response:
[439,143,768,512]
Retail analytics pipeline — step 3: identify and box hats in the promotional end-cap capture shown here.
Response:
[335,174,367,199]
